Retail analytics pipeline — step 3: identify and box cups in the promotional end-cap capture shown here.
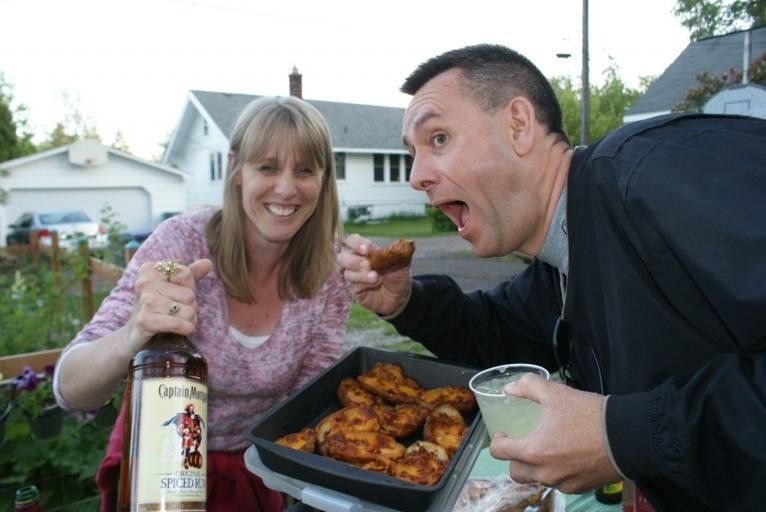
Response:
[469,364,552,442]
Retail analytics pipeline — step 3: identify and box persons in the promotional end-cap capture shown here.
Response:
[50,94,358,509]
[335,42,766,510]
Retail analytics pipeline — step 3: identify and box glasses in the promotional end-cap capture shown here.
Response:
[551,316,604,393]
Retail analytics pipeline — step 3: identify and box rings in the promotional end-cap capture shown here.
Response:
[167,303,182,316]
[153,257,182,283]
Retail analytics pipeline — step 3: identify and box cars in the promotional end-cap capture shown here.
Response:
[114,210,183,249]
[6,206,108,261]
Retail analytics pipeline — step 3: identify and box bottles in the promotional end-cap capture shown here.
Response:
[595,479,626,504]
[116,331,208,511]
[14,484,41,512]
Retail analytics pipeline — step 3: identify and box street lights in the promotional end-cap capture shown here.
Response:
[555,1,592,148]
